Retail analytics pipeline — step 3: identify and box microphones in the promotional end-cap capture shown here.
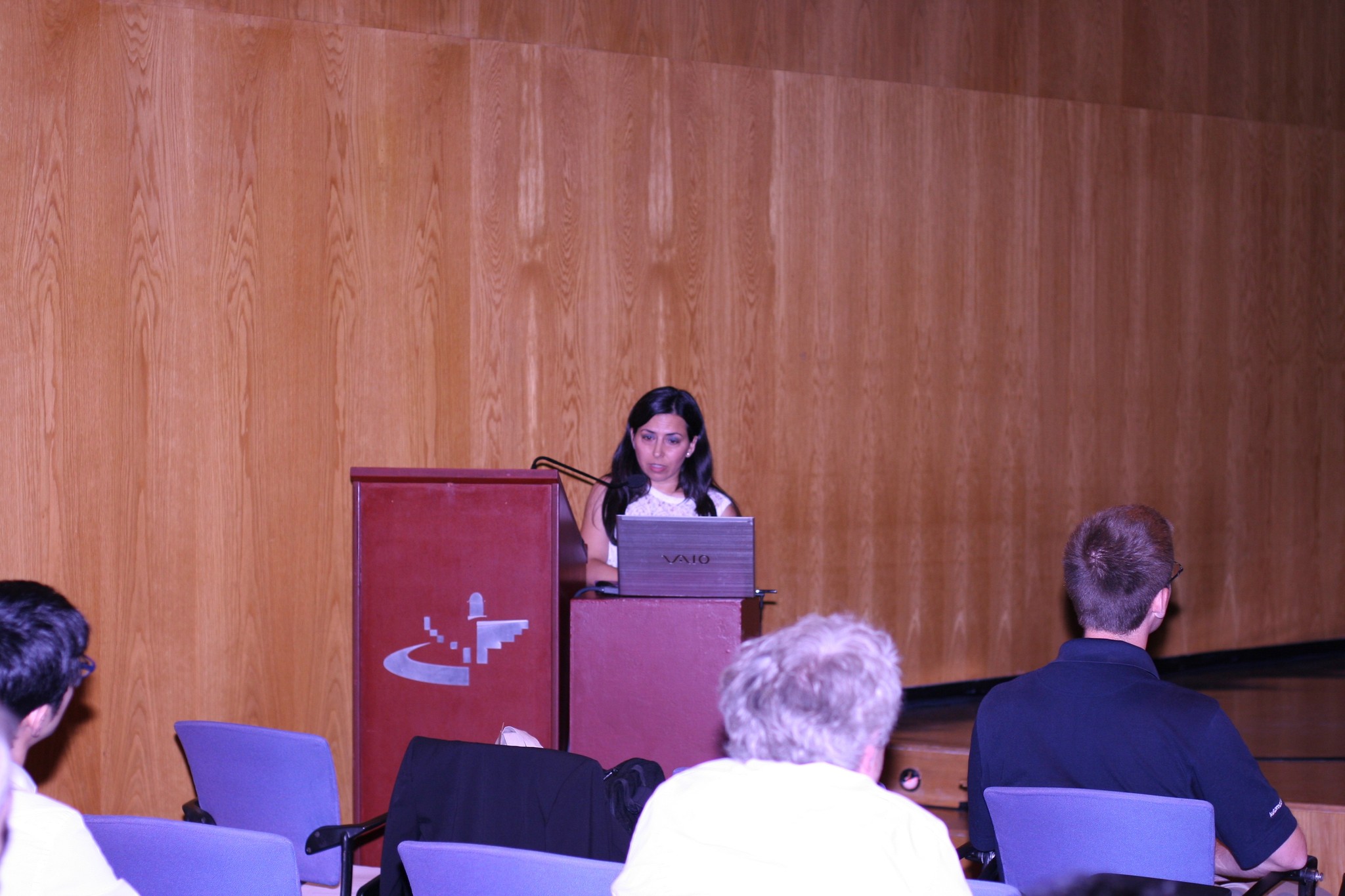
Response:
[611,473,647,491]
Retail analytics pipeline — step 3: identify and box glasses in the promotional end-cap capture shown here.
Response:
[78,654,95,678]
[1165,561,1183,587]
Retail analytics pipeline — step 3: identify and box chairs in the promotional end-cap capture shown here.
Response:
[172,720,391,896]
[337,733,623,896]
[982,786,1324,896]
[80,814,302,896]
[398,841,623,896]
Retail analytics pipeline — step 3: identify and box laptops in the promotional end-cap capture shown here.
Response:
[614,514,758,598]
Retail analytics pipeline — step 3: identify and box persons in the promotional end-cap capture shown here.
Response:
[1,578,141,890]
[608,613,976,896]
[966,505,1330,896]
[581,386,739,587]
